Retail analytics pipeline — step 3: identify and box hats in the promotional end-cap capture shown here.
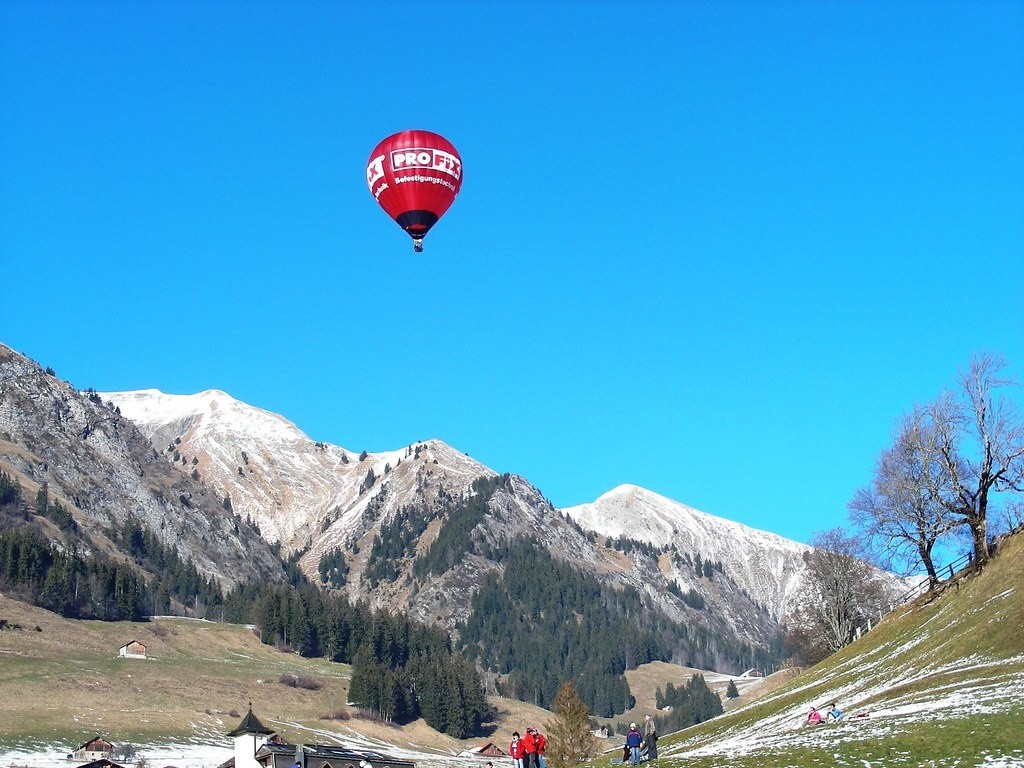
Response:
[527,728,532,733]
[630,723,636,728]
[533,728,537,732]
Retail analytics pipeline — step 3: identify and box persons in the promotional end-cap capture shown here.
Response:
[292,761,302,768]
[510,727,547,768]
[486,762,493,768]
[801,707,821,728]
[626,722,643,766]
[644,713,657,761]
[826,704,844,723]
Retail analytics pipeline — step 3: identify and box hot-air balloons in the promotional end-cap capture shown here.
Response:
[367,130,463,253]
